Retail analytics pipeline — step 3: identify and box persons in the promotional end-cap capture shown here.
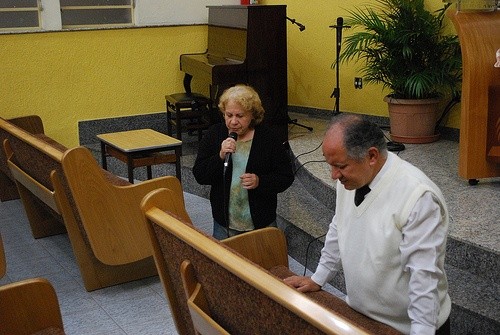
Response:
[282,112,452,335]
[192,84,295,240]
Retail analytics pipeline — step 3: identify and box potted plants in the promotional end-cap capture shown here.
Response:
[330,0,465,144]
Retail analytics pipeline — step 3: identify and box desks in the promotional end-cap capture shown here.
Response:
[95,129,183,186]
[447,8,500,186]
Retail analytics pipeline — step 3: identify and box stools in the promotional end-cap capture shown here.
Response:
[164,92,210,157]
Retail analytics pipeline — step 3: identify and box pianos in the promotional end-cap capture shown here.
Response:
[179,4,289,148]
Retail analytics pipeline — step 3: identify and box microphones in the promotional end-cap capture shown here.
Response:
[337,17,344,52]
[224,132,238,167]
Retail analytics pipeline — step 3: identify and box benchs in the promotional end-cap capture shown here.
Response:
[0,115,409,335]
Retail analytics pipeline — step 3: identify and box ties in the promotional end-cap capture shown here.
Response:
[354,184,371,207]
